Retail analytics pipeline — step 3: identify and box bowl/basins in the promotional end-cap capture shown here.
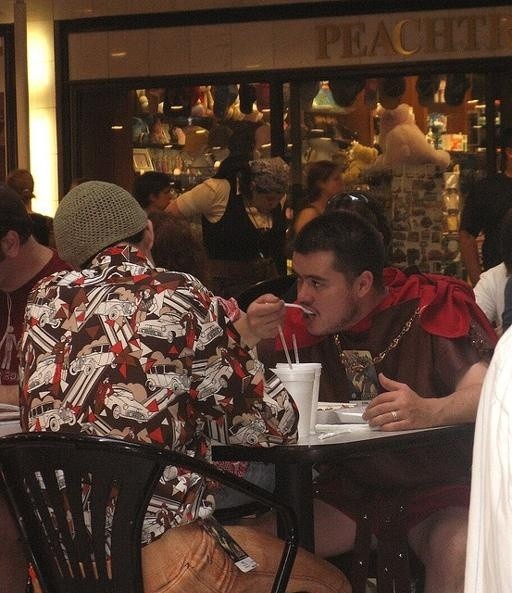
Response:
[316,396,372,424]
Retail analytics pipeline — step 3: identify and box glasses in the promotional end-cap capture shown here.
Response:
[256,227,270,257]
[327,191,369,205]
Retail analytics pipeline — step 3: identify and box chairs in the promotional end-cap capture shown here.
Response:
[0,428,302,592]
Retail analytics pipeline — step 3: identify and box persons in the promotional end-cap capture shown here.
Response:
[2,126,512,593]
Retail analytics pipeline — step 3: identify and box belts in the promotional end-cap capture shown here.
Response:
[195,515,258,574]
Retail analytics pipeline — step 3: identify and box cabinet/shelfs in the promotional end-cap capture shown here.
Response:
[133,110,259,182]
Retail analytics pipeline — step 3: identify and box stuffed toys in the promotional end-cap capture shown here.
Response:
[374,103,451,168]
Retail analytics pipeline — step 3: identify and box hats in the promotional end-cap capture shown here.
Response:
[299,79,320,109]
[256,81,270,113]
[239,83,256,112]
[211,83,237,117]
[52,181,148,268]
[241,155,289,191]
[329,76,366,106]
[415,73,440,106]
[145,85,201,118]
[184,123,270,160]
[444,70,470,104]
[378,76,405,109]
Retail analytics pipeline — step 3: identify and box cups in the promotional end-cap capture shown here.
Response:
[274,367,314,437]
[276,360,325,434]
